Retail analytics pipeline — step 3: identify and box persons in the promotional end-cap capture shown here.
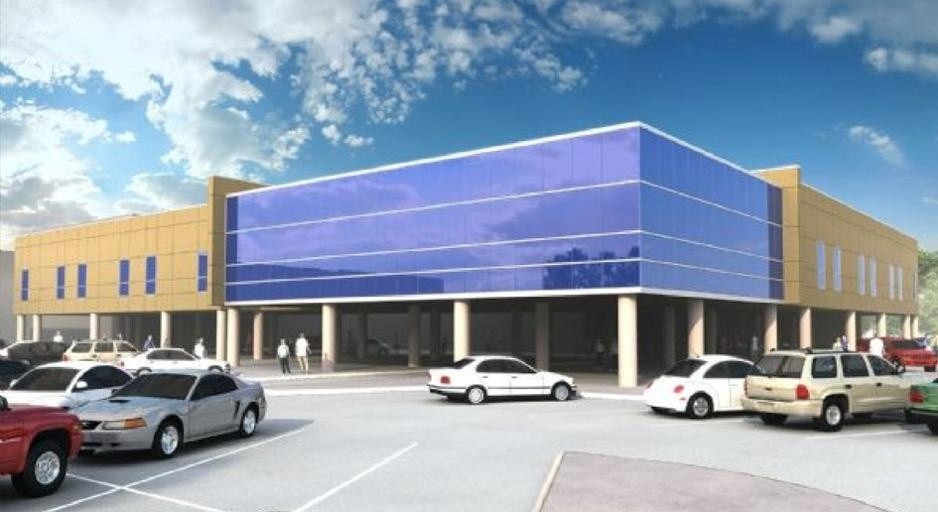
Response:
[831,335,842,350]
[52,326,159,352]
[590,336,606,355]
[192,336,209,361]
[923,332,938,350]
[294,332,312,373]
[868,332,888,359]
[275,338,291,376]
[840,328,848,350]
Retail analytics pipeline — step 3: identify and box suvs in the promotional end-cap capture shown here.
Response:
[739,345,927,431]
[0,392,83,496]
[61,338,140,363]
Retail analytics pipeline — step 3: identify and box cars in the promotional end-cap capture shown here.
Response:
[64,367,270,460]
[0,339,66,362]
[0,358,135,413]
[638,353,755,419]
[425,355,578,404]
[120,348,231,377]
[904,376,938,435]
[0,356,31,391]
[854,336,937,373]
